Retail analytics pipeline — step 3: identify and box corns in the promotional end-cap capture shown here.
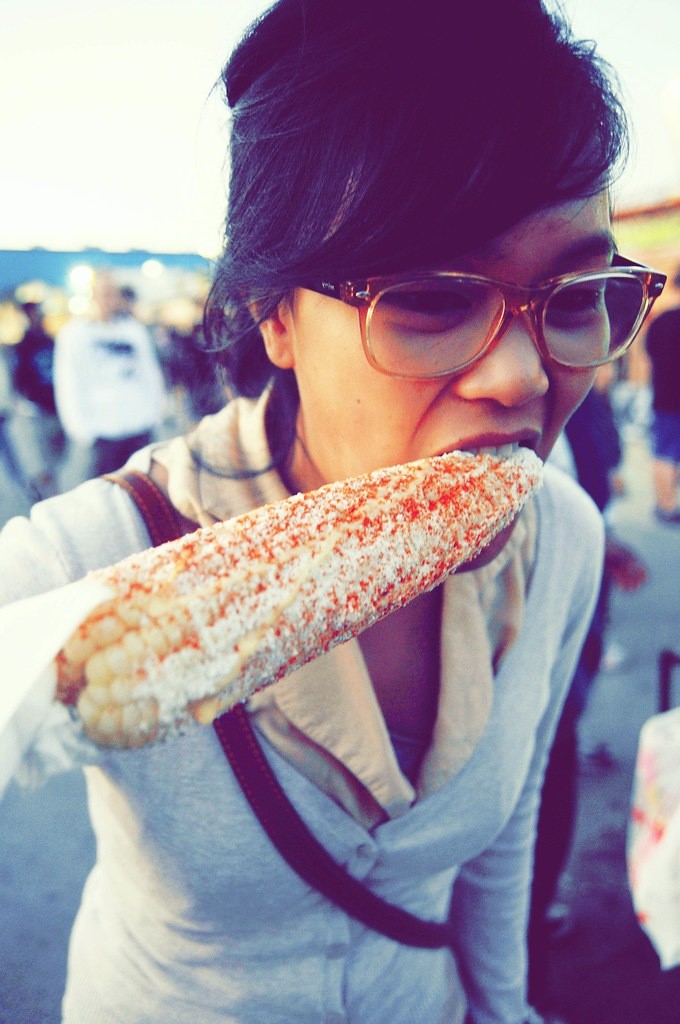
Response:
[51,446,547,750]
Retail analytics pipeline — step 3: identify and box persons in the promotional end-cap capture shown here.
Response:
[156,289,229,417]
[0,302,67,504]
[0,1,669,1024]
[50,272,166,477]
[646,271,680,523]
[530,388,623,936]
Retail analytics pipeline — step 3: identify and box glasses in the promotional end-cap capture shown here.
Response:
[296,251,668,383]
